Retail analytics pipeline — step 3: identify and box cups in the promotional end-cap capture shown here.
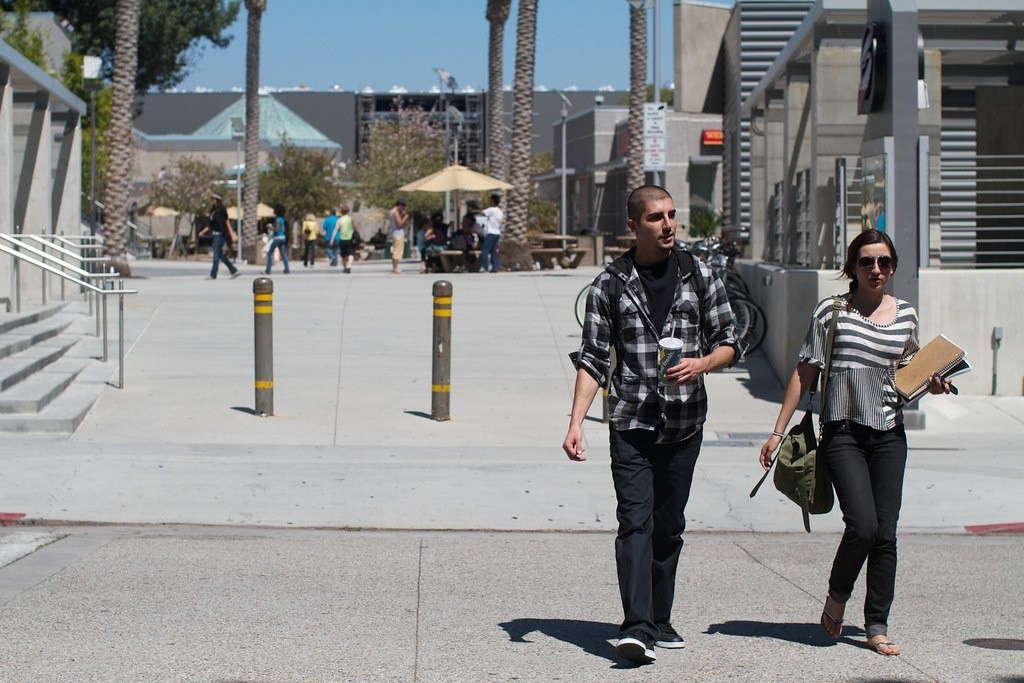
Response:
[658,337,684,385]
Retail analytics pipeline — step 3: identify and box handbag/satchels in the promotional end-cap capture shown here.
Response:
[773,409,834,513]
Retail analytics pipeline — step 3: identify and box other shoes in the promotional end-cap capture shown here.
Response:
[229,270,241,278]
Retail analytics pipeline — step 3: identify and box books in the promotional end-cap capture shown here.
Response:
[895,333,972,408]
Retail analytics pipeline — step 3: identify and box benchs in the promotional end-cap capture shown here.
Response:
[428,244,631,273]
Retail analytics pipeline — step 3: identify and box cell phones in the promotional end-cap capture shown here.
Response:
[932,373,958,394]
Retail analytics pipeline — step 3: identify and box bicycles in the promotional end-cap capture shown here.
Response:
[572,234,769,356]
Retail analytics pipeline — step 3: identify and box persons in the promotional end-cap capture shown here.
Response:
[302,213,318,267]
[759,228,953,656]
[322,210,341,267]
[329,205,358,273]
[562,185,742,665]
[479,194,504,273]
[389,199,409,274]
[370,228,392,259]
[198,192,241,279]
[415,200,484,273]
[260,208,289,274]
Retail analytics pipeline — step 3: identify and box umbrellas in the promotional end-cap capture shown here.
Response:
[227,202,275,220]
[364,209,390,220]
[399,164,515,230]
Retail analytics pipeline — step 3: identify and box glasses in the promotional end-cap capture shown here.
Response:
[855,255,894,268]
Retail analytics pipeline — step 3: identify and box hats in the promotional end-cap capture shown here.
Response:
[211,193,224,202]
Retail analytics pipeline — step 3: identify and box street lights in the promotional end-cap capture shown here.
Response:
[442,101,463,240]
[229,115,245,263]
[553,89,572,249]
[80,55,104,277]
[432,64,456,109]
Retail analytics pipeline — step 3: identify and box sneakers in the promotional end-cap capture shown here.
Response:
[654,623,685,648]
[616,629,656,665]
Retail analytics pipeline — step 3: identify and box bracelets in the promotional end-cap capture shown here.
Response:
[773,432,783,437]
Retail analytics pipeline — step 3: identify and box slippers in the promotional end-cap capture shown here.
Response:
[820,594,845,639]
[873,638,900,656]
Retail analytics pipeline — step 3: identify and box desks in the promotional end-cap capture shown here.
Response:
[536,233,578,270]
